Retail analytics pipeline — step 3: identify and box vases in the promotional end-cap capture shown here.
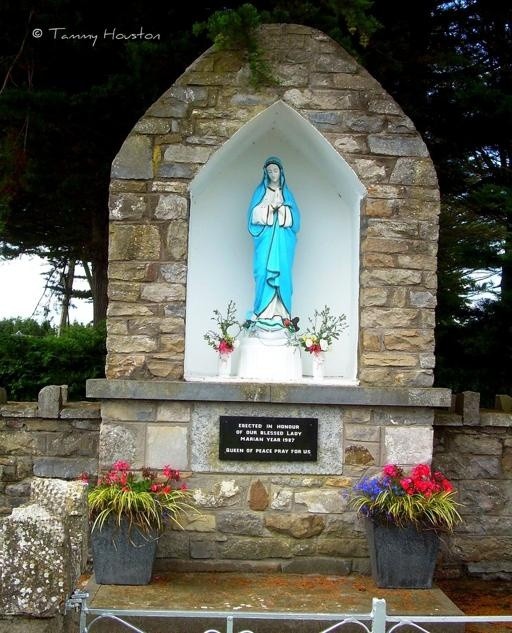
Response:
[364,516,439,588]
[300,348,318,377]
[89,513,158,586]
[217,340,241,376]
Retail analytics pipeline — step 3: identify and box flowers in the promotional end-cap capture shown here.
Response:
[339,461,466,535]
[283,305,349,357]
[79,460,203,550]
[203,300,244,354]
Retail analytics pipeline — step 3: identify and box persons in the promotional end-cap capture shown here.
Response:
[246,156,300,331]
